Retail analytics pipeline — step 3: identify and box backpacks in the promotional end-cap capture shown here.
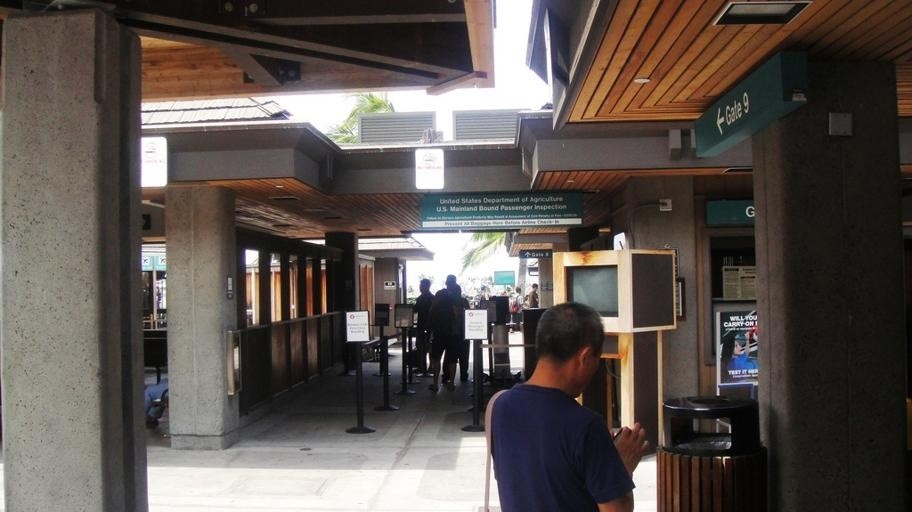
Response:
[512,298,519,313]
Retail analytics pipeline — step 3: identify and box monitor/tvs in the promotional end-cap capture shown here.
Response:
[569,265,618,317]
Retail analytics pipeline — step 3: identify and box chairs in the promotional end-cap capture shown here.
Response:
[140,388,168,427]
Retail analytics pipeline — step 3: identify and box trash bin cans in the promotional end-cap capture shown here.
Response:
[656,396,768,512]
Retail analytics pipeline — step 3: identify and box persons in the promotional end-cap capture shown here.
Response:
[145,377,169,429]
[405,275,538,393]
[485,302,650,512]
